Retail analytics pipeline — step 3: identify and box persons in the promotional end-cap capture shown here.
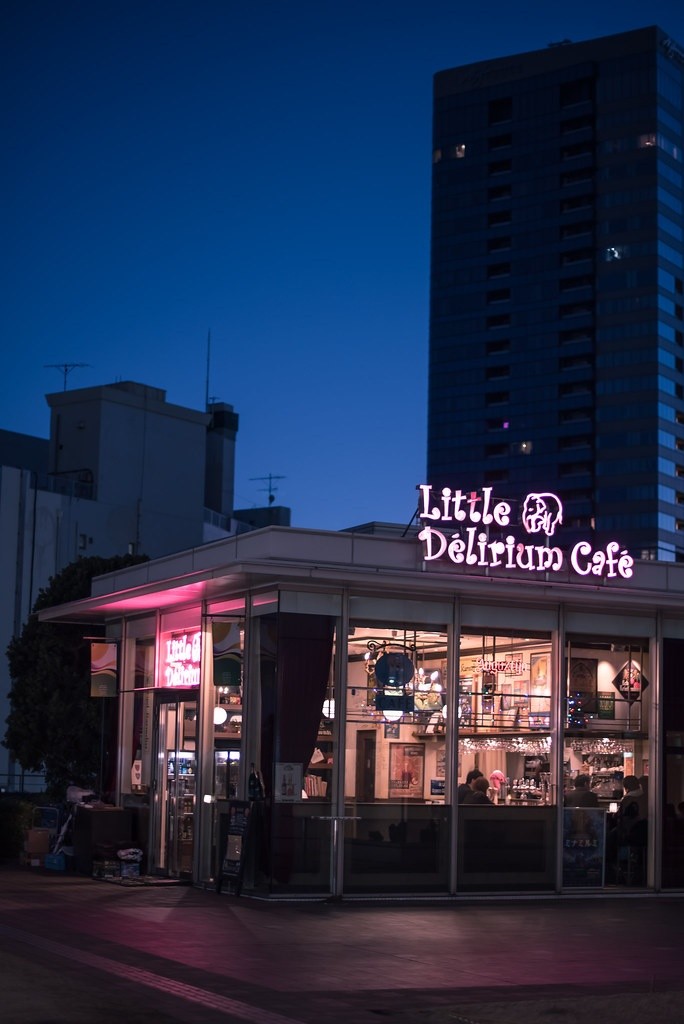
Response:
[604,774,647,872]
[458,769,484,804]
[564,774,599,808]
[462,777,495,805]
[637,776,648,795]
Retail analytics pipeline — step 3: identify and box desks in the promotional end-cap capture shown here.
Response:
[75,801,131,875]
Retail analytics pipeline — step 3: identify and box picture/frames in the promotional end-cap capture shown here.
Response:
[561,807,607,890]
[384,720,400,739]
[272,762,303,803]
[387,743,426,799]
[569,657,599,720]
[434,750,464,778]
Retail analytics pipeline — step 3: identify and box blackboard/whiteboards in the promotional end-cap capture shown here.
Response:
[219,799,256,881]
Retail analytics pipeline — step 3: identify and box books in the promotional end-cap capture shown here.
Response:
[304,774,328,798]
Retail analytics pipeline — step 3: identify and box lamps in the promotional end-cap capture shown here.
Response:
[364,630,444,723]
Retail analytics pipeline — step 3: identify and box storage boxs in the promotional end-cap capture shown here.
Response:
[121,861,141,880]
[19,829,73,872]
[92,858,121,878]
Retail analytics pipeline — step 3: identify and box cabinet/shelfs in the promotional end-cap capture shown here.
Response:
[211,649,340,804]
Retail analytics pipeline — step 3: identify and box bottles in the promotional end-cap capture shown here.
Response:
[249,762,264,801]
[281,774,294,795]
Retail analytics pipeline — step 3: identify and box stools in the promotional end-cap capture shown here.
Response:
[617,844,647,887]
